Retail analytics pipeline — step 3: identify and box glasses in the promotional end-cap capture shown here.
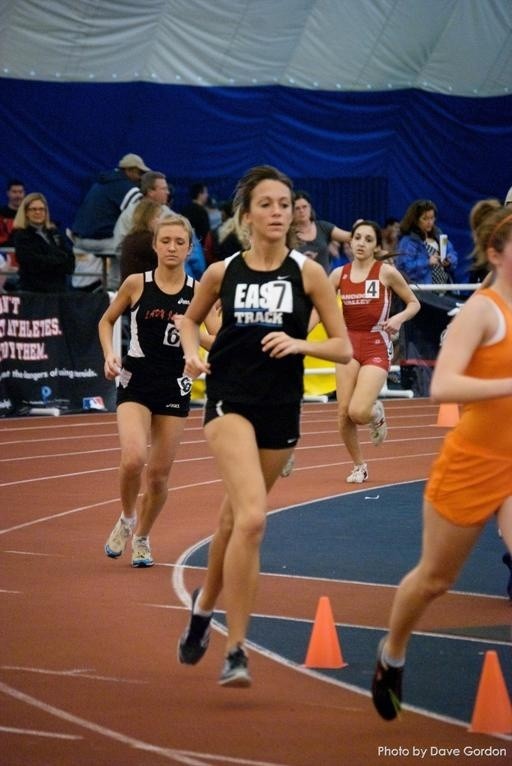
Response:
[26,207,45,212]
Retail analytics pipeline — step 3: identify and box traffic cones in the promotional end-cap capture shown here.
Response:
[294,591,356,678]
[427,398,465,427]
[463,650,512,739]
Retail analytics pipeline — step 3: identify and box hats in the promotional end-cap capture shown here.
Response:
[117,152,153,173]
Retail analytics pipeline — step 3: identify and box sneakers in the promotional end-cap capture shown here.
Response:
[131,534,156,569]
[369,400,388,447]
[345,464,369,484]
[216,646,252,690]
[104,510,138,558]
[175,586,211,667]
[279,453,295,478]
[368,632,406,724]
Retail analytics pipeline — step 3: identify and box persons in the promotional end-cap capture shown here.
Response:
[369,196,511,726]
[304,219,424,486]
[94,210,221,572]
[214,203,321,478]
[175,161,354,693]
